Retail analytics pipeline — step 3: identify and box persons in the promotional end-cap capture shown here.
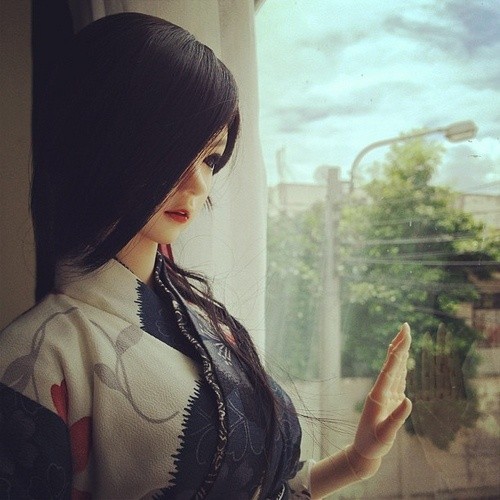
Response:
[405,252,500,500]
[0,12,411,500]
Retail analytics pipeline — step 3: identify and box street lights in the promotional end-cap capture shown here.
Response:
[318,119,478,500]
